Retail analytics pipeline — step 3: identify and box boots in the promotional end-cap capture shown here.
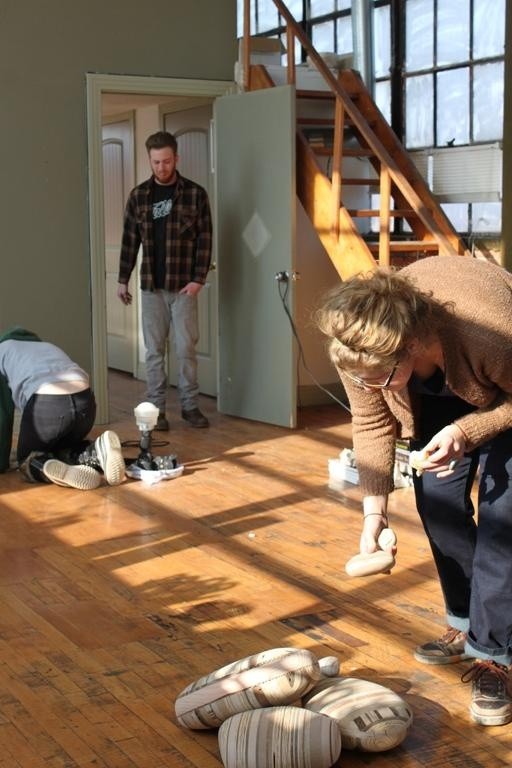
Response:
[80,430,126,485]
[24,452,101,492]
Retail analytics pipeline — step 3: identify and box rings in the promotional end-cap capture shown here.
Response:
[447,457,461,468]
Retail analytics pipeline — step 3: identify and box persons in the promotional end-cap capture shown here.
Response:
[117,132,214,433]
[1,328,126,491]
[313,255,512,725]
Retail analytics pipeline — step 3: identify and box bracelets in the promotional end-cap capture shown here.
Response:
[361,511,387,519]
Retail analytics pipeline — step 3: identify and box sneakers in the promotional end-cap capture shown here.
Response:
[461,660,512,725]
[413,628,470,666]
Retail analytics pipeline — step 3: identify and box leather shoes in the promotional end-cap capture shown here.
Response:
[182,407,207,428]
[154,415,170,432]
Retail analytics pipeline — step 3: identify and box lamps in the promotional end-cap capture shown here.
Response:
[133,402,159,470]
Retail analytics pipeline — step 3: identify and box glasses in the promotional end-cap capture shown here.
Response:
[337,348,409,389]
